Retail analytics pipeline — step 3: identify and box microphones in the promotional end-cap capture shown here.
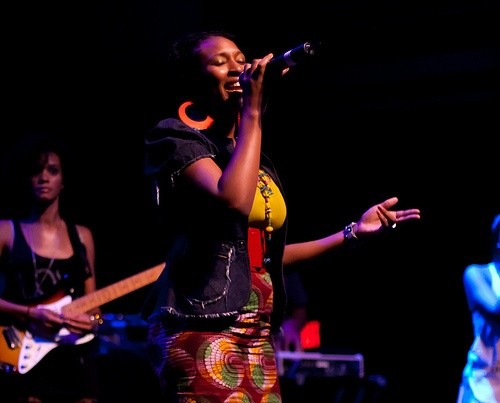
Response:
[238,42,315,85]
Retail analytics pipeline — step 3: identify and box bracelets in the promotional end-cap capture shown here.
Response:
[26,306,30,318]
[345,222,358,240]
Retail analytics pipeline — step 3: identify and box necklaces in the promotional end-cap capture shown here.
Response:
[256,169,273,240]
[29,221,62,294]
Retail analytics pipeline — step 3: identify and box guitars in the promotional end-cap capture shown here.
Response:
[0,262,167,375]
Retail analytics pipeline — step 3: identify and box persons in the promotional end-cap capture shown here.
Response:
[144,30,421,403]
[280,307,307,353]
[0,144,103,403]
[458,215,500,402]
